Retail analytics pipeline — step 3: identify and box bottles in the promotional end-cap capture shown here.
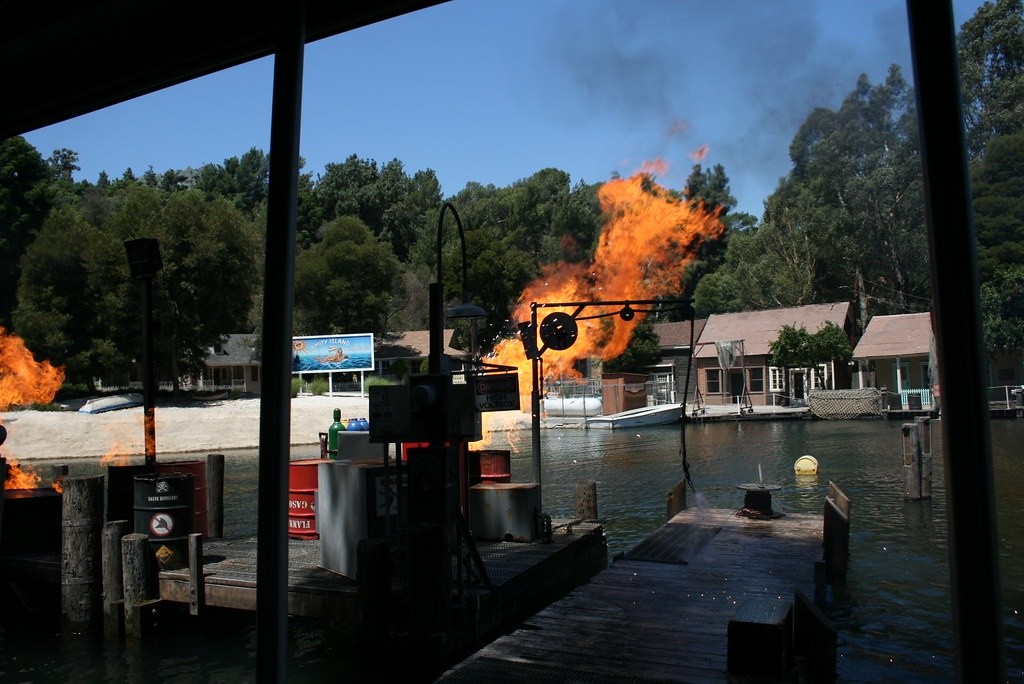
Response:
[340,418,369,431]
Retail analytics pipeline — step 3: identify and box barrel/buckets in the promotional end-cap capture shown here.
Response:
[134,472,196,571]
[159,461,208,538]
[794,454,818,475]
[470,450,511,486]
[288,459,334,540]
[107,465,148,533]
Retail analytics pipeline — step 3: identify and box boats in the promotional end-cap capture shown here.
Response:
[587,402,684,430]
[78,393,145,415]
